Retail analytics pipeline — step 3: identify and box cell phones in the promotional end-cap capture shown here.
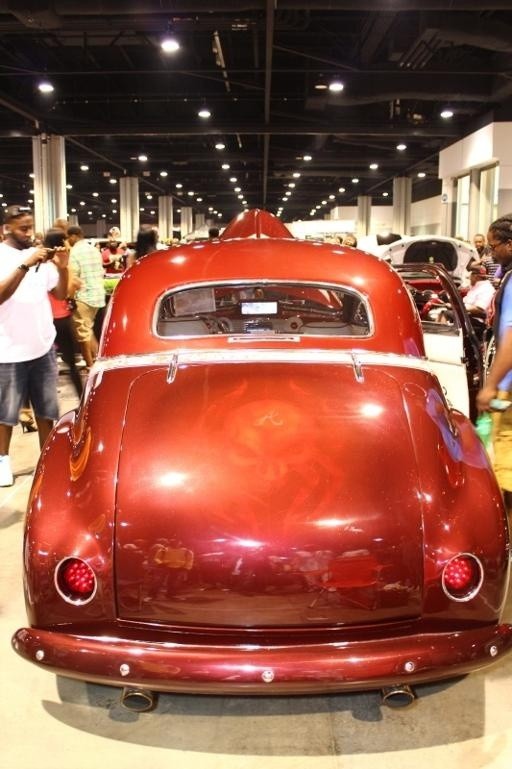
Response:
[489,398,512,413]
[34,249,56,272]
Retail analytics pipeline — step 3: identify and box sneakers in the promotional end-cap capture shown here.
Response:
[0,455,14,487]
[79,366,90,375]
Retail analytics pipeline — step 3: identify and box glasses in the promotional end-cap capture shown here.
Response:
[487,239,508,252]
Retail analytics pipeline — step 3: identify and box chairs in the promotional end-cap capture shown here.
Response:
[297,321,351,334]
[159,315,210,335]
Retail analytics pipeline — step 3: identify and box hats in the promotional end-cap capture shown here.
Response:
[471,266,492,278]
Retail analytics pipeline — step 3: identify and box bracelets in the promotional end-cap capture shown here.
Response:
[17,263,30,274]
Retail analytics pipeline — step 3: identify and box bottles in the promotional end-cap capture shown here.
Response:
[475,410,494,450]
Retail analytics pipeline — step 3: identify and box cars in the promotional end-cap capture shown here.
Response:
[9,208,510,715]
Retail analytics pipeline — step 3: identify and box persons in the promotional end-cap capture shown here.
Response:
[1,204,72,487]
[475,214,511,490]
[465,234,492,286]
[36,218,184,408]
[441,268,496,340]
[208,228,219,238]
